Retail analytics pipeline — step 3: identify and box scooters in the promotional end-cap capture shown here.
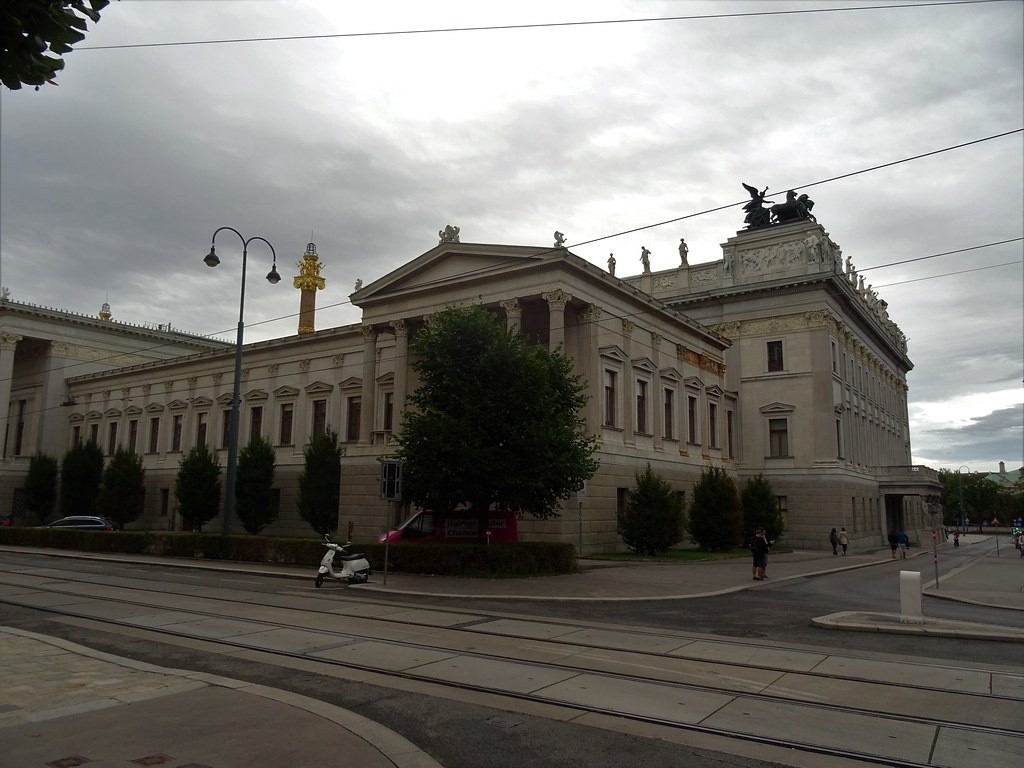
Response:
[314,534,371,588]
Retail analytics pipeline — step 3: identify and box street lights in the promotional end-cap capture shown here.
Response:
[203,226,282,555]
[958,466,971,537]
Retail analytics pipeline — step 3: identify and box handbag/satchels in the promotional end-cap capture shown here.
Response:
[839,538,841,545]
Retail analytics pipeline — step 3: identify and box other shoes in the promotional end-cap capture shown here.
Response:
[833,551,837,555]
[762,575,769,578]
[893,556,897,558]
[753,577,760,580]
[901,556,903,558]
[760,577,765,581]
[842,552,844,556]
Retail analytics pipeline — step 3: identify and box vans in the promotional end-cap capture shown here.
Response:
[377,507,521,545]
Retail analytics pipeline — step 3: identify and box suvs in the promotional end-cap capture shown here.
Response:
[36,516,122,532]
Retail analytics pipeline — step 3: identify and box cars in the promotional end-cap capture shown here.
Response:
[0,514,13,527]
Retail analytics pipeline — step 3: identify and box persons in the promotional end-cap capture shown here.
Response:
[830,527,850,556]
[954,531,960,548]
[759,185,775,207]
[678,238,688,265]
[749,526,775,581]
[888,527,909,560]
[1015,530,1024,558]
[640,246,652,273]
[846,255,910,356]
[607,253,616,276]
